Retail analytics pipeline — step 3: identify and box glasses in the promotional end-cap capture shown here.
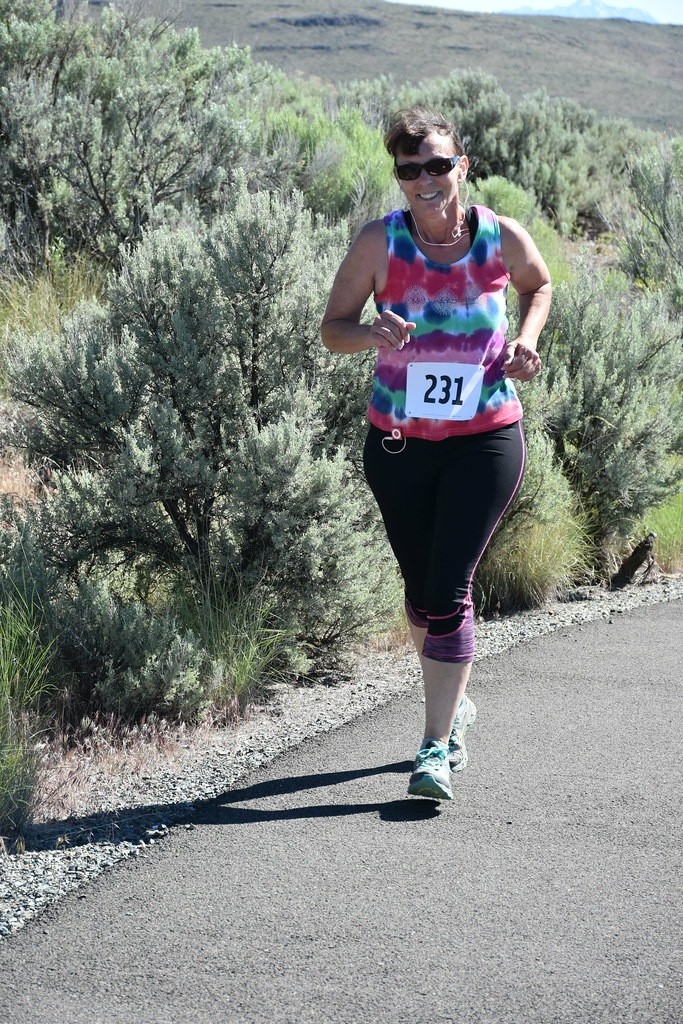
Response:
[394,155,460,180]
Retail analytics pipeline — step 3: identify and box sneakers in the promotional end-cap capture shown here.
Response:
[407,741,453,801]
[448,694,476,773]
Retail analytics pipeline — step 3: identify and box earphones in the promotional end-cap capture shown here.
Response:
[459,168,463,179]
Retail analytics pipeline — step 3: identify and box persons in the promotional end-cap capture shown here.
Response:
[320,109,552,798]
